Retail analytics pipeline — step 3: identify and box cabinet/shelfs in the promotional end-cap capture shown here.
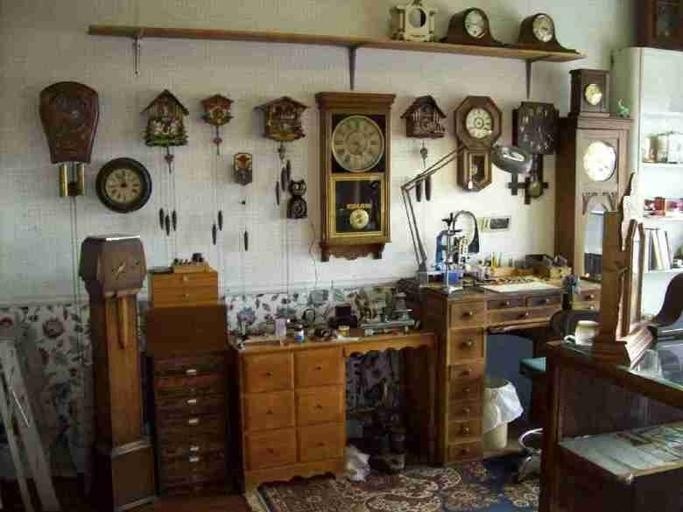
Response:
[400,276,602,472]
[143,353,237,499]
[610,46,683,326]
[229,328,438,498]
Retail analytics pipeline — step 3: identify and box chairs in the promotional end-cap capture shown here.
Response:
[511,307,599,486]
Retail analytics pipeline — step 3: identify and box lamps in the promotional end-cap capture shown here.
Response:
[399,143,535,279]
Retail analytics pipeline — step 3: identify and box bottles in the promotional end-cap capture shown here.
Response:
[294,324,304,342]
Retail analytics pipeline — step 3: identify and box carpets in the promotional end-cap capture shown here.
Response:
[243,451,541,512]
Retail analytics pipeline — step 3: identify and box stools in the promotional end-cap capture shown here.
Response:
[552,419,683,511]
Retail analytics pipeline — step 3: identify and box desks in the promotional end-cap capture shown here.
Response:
[536,330,682,511]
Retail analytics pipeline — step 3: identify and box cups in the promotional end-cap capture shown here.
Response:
[575,320,598,347]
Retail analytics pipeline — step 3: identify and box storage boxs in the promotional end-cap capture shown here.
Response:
[145,265,220,308]
[139,298,228,356]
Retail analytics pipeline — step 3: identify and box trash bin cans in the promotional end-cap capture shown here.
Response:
[481,378,511,449]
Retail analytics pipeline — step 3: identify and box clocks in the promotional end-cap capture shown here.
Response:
[464,106,494,139]
[583,141,618,182]
[104,167,143,205]
[464,9,488,37]
[332,115,385,174]
[584,83,602,106]
[531,13,554,43]
